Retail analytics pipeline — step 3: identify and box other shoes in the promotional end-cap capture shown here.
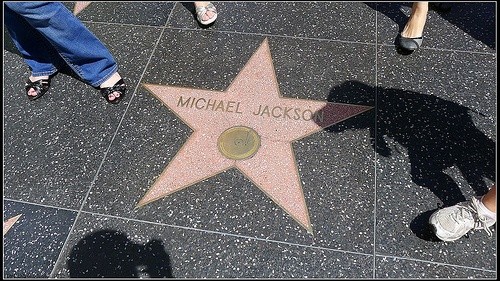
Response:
[401,24,423,51]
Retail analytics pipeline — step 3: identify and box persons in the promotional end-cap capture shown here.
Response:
[4,1,126,104]
[428,183,497,242]
[398,1,430,52]
[194,0,218,25]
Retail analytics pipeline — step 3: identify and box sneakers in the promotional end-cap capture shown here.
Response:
[429,195,496,242]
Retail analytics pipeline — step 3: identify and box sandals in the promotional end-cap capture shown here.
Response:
[98,72,127,104]
[195,3,218,25]
[25,75,49,102]
